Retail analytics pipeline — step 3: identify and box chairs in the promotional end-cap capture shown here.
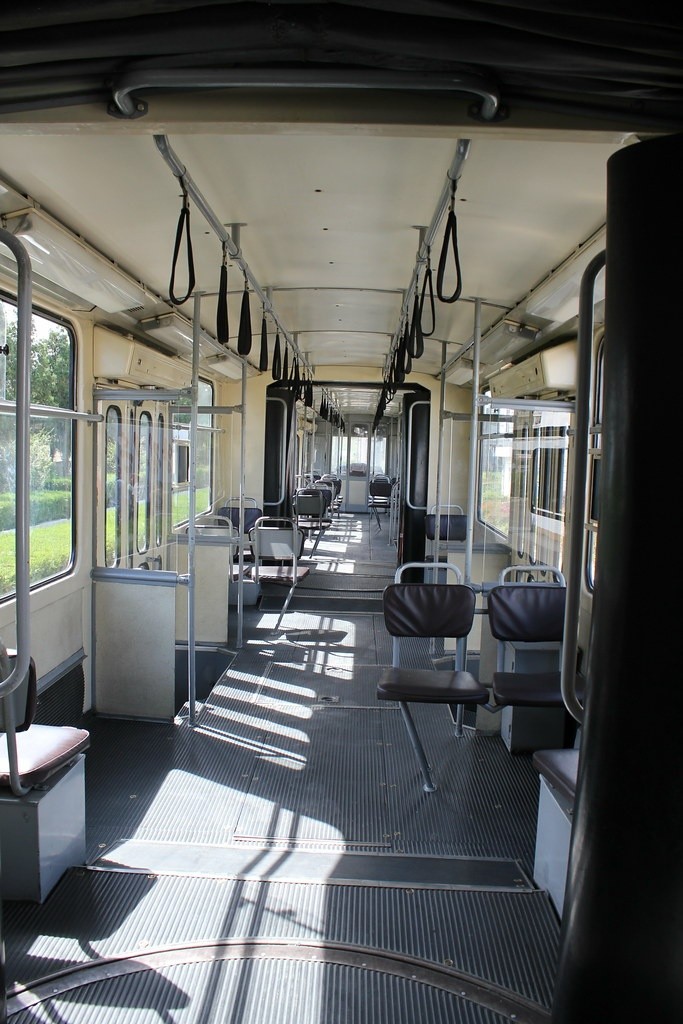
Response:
[374,557,576,794]
[185,498,309,637]
[295,473,343,541]
[0,646,94,902]
[424,505,467,586]
[368,474,400,534]
